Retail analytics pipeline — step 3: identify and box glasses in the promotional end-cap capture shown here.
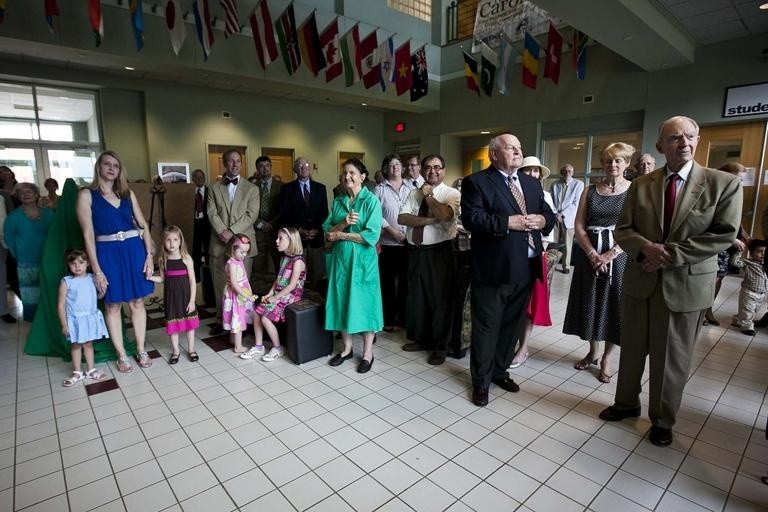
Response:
[423,166,444,172]
[296,162,310,168]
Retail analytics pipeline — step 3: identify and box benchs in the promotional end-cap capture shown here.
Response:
[246,270,334,365]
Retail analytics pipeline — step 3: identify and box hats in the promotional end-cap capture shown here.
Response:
[517,155,551,181]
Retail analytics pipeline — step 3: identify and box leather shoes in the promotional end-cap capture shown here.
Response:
[740,328,757,337]
[492,375,521,393]
[188,351,199,363]
[356,353,375,374]
[329,348,354,366]
[759,475,768,486]
[169,348,181,365]
[730,322,742,328]
[401,339,433,352]
[648,423,672,447]
[472,385,488,406]
[116,357,132,374]
[508,348,530,370]
[137,352,153,368]
[208,322,224,335]
[704,314,721,327]
[427,349,446,366]
[2,314,16,324]
[597,403,642,422]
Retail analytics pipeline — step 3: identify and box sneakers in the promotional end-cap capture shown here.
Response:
[240,345,266,359]
[261,346,286,362]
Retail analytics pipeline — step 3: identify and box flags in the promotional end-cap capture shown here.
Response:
[44,0,60,36]
[128,0,145,52]
[88,0,104,48]
[191,0,429,102]
[0,0,6,23]
[162,0,187,56]
[463,22,588,98]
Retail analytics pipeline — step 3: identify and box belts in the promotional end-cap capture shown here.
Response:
[408,241,454,250]
[96,230,139,241]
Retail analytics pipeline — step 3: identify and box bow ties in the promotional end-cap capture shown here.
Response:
[223,178,238,186]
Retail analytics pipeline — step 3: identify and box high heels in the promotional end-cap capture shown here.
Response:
[598,359,613,383]
[575,349,600,370]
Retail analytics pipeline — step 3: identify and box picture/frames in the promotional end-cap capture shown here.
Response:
[156,160,192,185]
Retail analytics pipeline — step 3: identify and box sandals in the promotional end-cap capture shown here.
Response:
[63,371,85,387]
[84,368,108,381]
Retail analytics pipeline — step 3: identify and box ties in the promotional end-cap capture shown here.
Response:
[412,181,417,188]
[196,188,204,217]
[303,184,310,210]
[260,182,270,221]
[507,175,537,250]
[561,185,567,204]
[665,174,681,238]
[410,196,429,248]
